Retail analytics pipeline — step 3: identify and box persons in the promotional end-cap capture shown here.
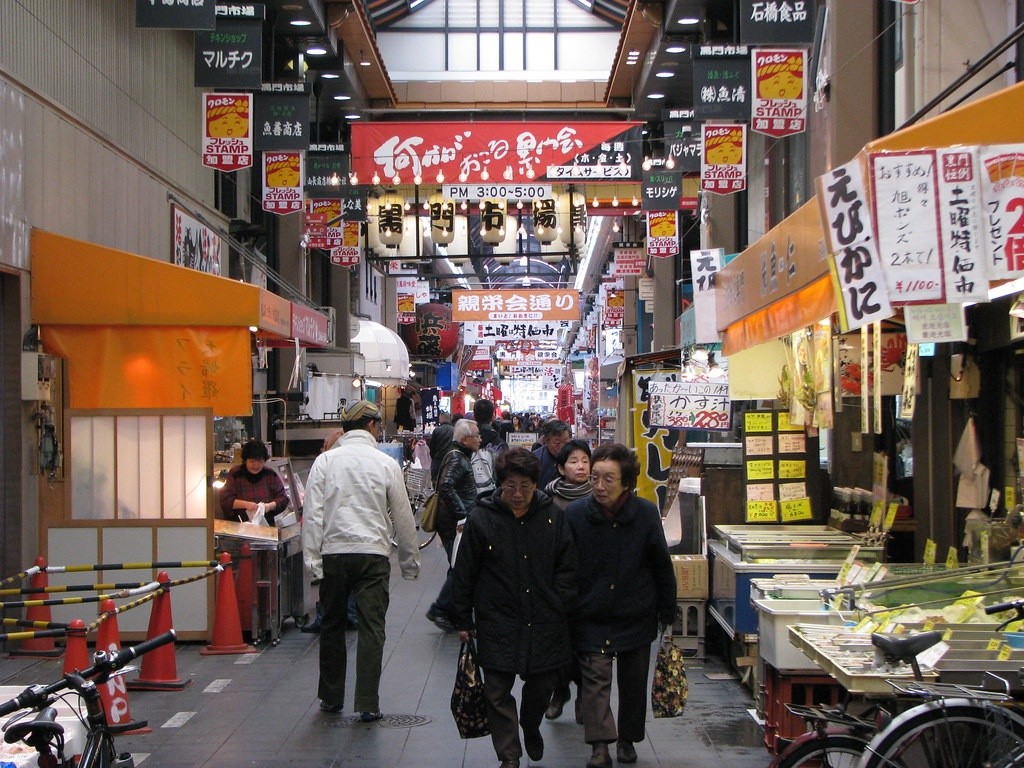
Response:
[564,443,677,768]
[219,440,289,528]
[448,447,579,768]
[394,390,594,726]
[301,431,358,632]
[300,400,421,721]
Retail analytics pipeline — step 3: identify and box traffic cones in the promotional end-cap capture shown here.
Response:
[61,619,102,712]
[125,570,194,691]
[0,557,66,661]
[90,599,153,735]
[199,543,260,656]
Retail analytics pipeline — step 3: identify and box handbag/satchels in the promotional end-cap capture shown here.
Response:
[451,635,490,739]
[421,492,438,532]
[651,630,687,717]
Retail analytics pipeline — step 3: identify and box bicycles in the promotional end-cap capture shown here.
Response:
[0,629,181,768]
[768,599,1024,768]
[387,455,438,550]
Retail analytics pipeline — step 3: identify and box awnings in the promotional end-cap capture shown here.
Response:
[351,320,409,388]
[714,81,1024,352]
[28,226,328,348]
[616,348,681,381]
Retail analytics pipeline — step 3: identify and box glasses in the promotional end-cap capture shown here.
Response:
[585,473,623,488]
[500,481,535,496]
[466,434,481,441]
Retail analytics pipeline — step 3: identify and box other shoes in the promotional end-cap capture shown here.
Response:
[499,760,520,768]
[519,721,544,761]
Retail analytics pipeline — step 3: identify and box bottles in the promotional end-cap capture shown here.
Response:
[830,487,873,522]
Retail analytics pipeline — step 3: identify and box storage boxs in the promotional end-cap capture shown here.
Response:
[671,554,709,599]
[747,577,863,768]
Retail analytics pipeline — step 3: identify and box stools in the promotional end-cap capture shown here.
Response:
[670,600,707,666]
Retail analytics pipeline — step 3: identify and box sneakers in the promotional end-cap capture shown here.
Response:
[544,688,571,720]
[457,619,477,637]
[616,741,637,762]
[320,701,343,713]
[360,710,384,722]
[427,602,455,635]
[588,752,612,768]
[574,698,586,725]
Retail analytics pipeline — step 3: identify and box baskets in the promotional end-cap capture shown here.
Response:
[406,467,430,493]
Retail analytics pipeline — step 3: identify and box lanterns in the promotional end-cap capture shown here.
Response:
[364,189,423,267]
[492,215,517,266]
[446,215,469,266]
[532,184,588,262]
[479,198,507,246]
[430,189,455,248]
[402,300,458,365]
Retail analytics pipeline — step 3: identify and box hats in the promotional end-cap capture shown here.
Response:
[341,399,382,423]
